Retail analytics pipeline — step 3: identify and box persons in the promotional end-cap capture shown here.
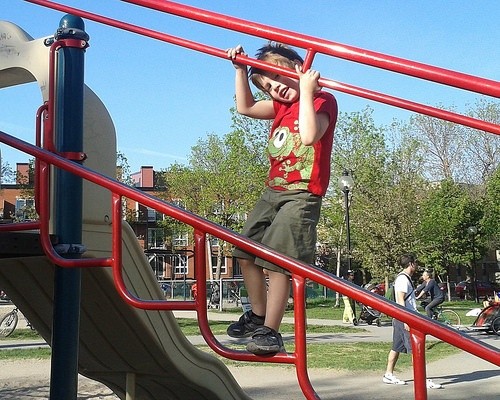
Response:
[382,253,442,389]
[464,273,474,301]
[413,268,446,320]
[224,42,339,355]
[211,281,220,309]
[340,269,359,326]
[191,281,198,300]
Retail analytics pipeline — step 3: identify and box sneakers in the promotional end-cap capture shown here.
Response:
[227,309,264,338]
[246,326,285,354]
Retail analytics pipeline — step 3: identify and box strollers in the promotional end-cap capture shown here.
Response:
[353,282,385,327]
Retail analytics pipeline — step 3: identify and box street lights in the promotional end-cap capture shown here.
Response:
[337,168,355,316]
[467,226,479,304]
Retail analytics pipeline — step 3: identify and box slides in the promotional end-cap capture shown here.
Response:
[0,221,252,400]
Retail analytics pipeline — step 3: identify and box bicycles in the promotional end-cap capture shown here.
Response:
[415,298,461,329]
[0,306,36,337]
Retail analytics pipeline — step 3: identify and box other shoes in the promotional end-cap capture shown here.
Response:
[382,374,405,385]
[425,379,442,389]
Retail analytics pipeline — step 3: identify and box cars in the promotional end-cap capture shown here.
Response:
[455,279,499,300]
